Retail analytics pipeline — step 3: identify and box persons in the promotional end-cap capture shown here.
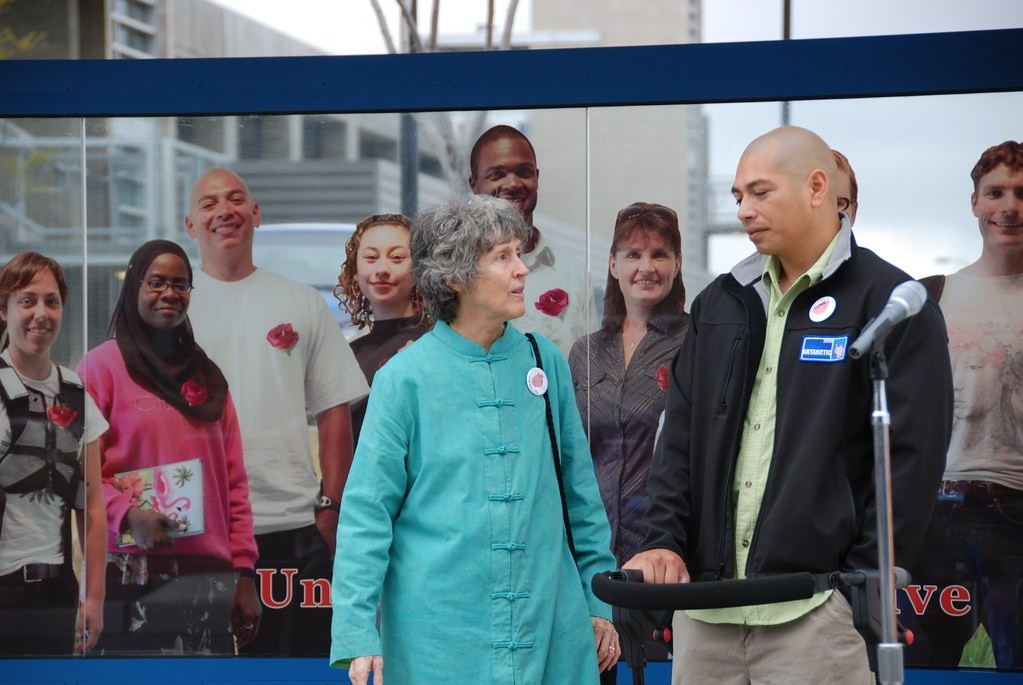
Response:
[329,181,622,685]
[621,125,953,685]
[470,125,598,363]
[0,251,109,657]
[567,201,690,568]
[184,169,370,657]
[74,240,263,656]
[902,139,1023,672]
[831,150,857,228]
[335,212,433,454]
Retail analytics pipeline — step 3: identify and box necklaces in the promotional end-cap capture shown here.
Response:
[627,325,646,349]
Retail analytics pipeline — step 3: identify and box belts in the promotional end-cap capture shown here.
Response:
[938,479,1016,498]
[13,564,62,583]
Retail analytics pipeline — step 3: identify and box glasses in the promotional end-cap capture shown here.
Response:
[837,197,855,210]
[139,279,195,294]
[615,205,679,231]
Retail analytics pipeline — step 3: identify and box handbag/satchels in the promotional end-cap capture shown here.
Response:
[596,648,617,685]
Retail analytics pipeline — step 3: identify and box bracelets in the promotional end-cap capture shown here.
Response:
[317,496,340,513]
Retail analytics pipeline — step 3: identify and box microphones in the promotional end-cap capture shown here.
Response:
[849,280,927,358]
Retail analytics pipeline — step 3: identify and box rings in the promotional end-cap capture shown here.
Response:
[608,647,614,650]
[244,623,254,632]
